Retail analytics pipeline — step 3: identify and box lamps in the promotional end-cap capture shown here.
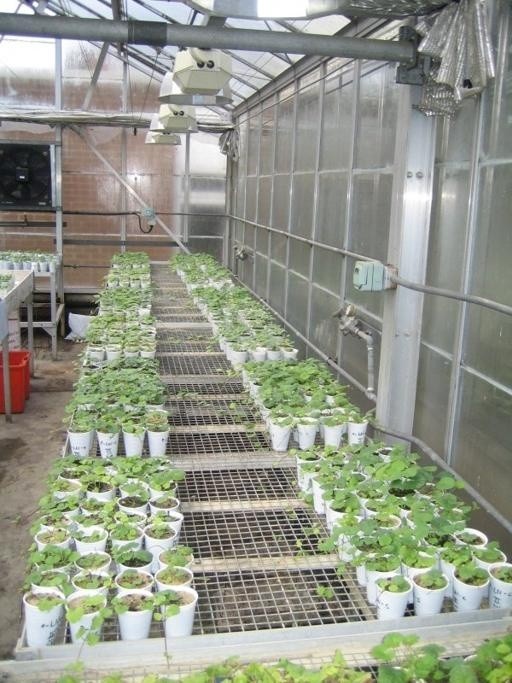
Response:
[142,44,239,149]
